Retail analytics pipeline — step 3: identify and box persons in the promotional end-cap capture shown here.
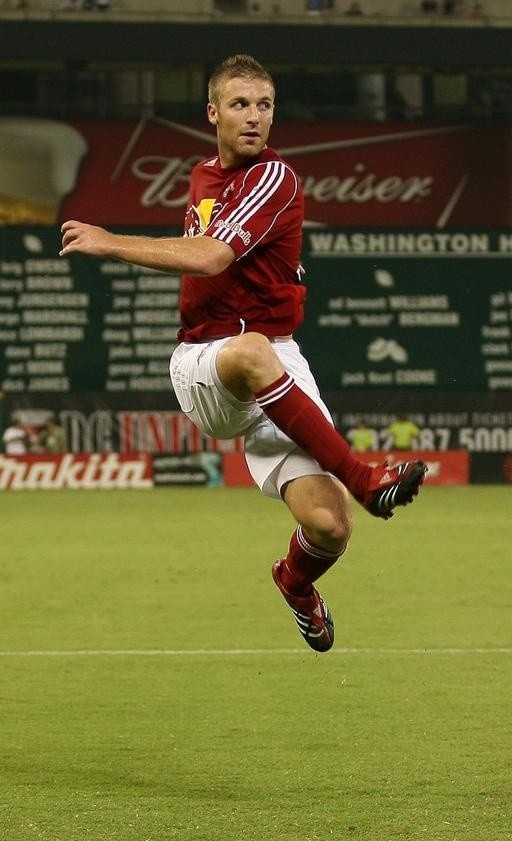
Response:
[55,49,426,653]
[385,408,424,453]
[40,413,68,454]
[346,416,380,452]
[2,417,30,455]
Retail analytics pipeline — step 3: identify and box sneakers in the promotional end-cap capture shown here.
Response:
[362,461,428,521]
[271,558,336,652]
